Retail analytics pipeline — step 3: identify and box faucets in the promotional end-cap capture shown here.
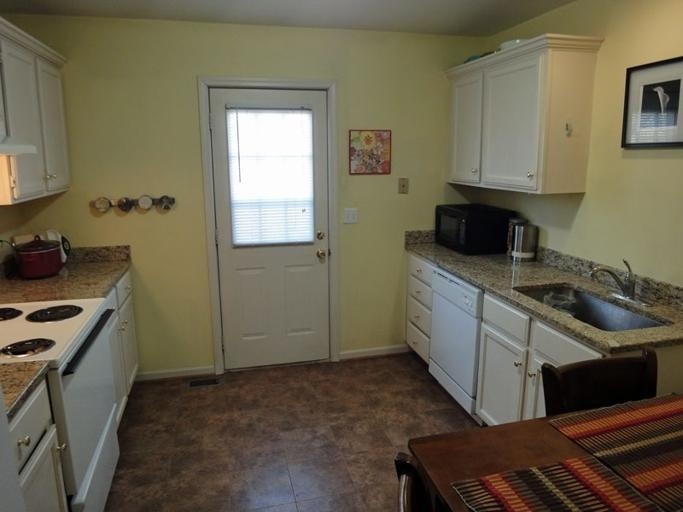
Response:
[589,257,636,299]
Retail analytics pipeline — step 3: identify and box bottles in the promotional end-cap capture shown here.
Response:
[505,218,527,254]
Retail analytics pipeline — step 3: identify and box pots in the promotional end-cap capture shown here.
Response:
[1,235,62,279]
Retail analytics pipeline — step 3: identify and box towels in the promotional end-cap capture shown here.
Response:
[450,455,666,512]
[548,392,683,512]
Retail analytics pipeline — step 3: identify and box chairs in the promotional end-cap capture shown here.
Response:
[541,346,658,416]
[394,451,431,512]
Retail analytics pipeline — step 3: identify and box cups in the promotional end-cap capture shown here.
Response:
[512,224,537,262]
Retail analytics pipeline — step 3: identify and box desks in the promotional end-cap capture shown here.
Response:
[407,392,683,512]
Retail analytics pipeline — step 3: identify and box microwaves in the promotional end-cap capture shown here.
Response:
[434,203,516,254]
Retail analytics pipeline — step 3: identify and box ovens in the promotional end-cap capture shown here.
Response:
[44,308,122,512]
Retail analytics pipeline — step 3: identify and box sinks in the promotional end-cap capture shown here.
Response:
[512,282,675,332]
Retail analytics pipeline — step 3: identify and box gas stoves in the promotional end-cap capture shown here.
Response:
[0,298,106,359]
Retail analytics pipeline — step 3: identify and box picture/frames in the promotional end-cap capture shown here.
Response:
[349,129,392,175]
[621,56,683,148]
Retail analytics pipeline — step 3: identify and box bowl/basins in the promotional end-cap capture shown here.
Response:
[543,295,576,317]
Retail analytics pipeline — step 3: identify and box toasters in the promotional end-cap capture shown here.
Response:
[11,227,70,265]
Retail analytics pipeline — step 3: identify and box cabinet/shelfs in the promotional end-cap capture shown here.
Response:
[475,293,683,427]
[0,35,70,206]
[8,379,68,512]
[106,271,139,433]
[442,33,605,195]
[405,252,435,365]
